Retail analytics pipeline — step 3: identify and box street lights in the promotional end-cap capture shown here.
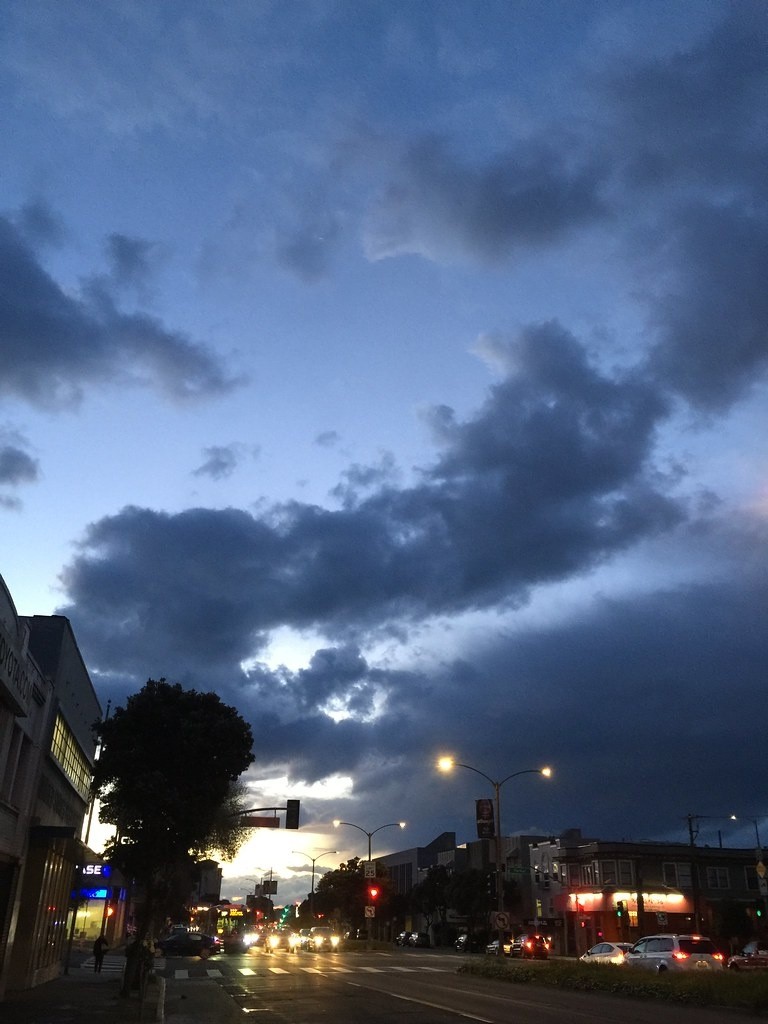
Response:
[333,818,406,951]
[436,755,552,962]
[290,850,336,927]
[731,814,763,862]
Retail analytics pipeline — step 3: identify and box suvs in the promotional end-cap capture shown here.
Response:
[510,934,548,959]
[409,932,430,948]
[306,926,338,952]
[453,932,483,952]
[624,933,725,976]
[396,930,412,947]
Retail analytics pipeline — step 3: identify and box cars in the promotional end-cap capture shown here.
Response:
[153,931,221,959]
[728,940,768,971]
[579,941,633,964]
[486,938,512,955]
[344,928,367,940]
[245,924,310,953]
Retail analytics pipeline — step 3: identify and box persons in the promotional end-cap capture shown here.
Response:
[93,934,109,973]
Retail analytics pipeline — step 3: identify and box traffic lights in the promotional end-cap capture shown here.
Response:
[369,886,377,898]
[597,930,603,937]
[617,901,623,917]
[756,900,762,918]
